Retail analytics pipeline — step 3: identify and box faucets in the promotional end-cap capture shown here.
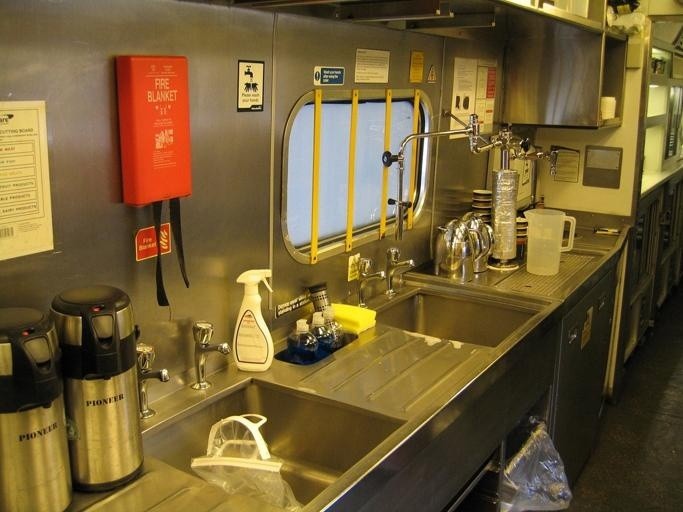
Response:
[358,257,386,308]
[386,247,416,295]
[192,320,231,390]
[137,343,172,418]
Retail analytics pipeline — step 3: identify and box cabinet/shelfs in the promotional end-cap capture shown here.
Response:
[625,38,683,359]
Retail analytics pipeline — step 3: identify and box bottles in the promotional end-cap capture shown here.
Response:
[599,96,616,120]
[293,306,345,358]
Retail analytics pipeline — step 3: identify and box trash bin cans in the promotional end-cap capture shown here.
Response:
[486,414,574,512]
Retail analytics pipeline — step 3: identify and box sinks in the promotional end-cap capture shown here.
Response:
[140,377,407,512]
[375,288,541,347]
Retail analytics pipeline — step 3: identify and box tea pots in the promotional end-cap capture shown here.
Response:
[433,211,492,282]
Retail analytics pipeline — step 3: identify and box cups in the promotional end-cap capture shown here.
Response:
[523,208,577,276]
[470,189,493,225]
[516,217,527,257]
